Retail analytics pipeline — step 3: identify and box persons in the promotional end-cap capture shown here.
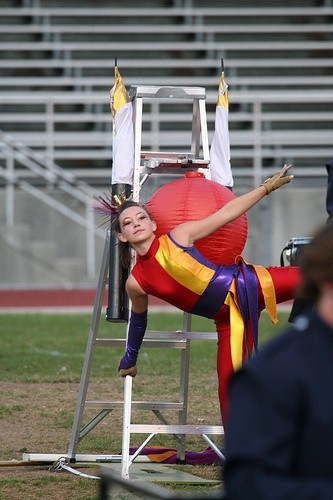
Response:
[222,224,333,500]
[89,162,318,473]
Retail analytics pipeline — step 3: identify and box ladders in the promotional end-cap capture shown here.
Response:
[65,84,212,482]
